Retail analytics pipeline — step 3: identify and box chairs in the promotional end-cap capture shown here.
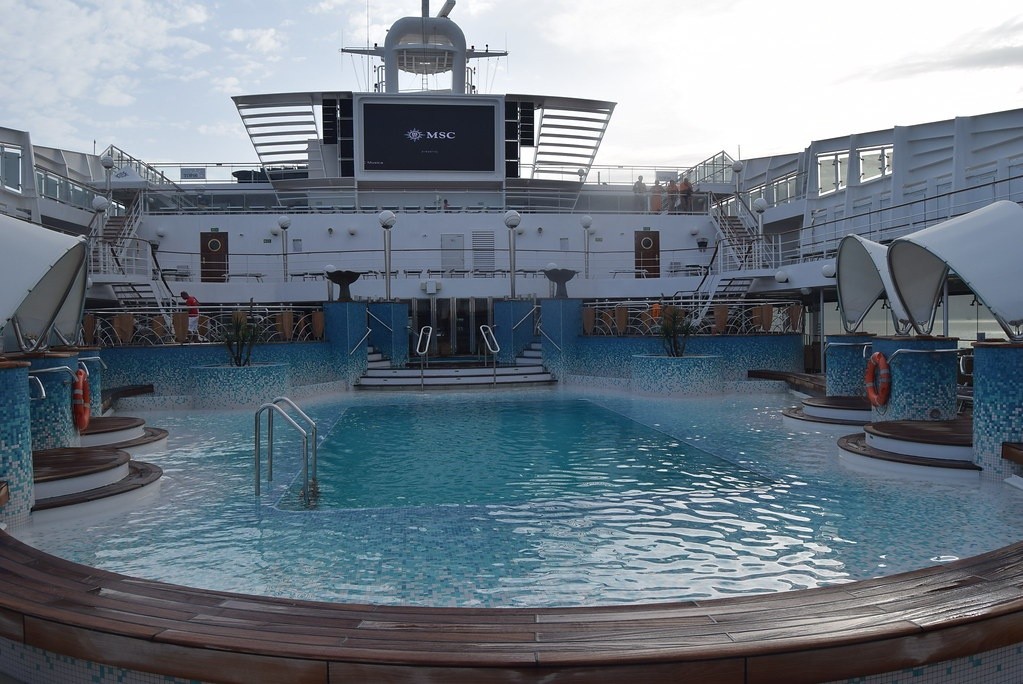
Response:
[289,268,580,282]
[159,205,524,214]
[174,265,193,282]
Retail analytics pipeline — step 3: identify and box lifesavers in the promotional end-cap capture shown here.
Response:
[73,368,91,430]
[865,351,890,407]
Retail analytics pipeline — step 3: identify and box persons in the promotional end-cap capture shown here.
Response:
[666,179,679,214]
[649,180,665,215]
[679,178,692,215]
[633,176,646,215]
[181,292,199,342]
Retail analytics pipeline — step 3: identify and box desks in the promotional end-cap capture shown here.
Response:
[222,273,267,283]
[665,268,707,278]
[610,269,648,279]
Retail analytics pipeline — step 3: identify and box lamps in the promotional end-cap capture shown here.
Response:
[347,225,357,237]
[155,226,166,238]
[822,264,835,277]
[774,271,789,283]
[270,226,280,236]
[689,226,699,235]
[517,226,525,235]
